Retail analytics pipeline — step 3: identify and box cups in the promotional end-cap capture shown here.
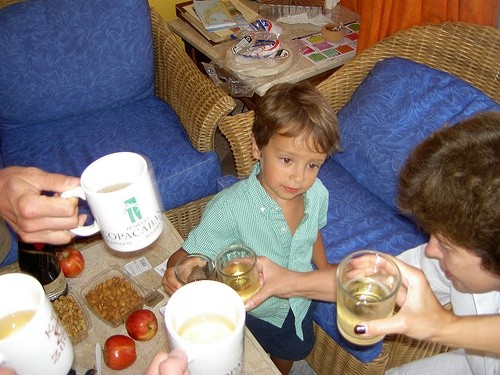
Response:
[164,279,246,375]
[59,150,164,253]
[0,272,75,375]
[174,253,217,285]
[336,249,403,348]
[215,244,262,303]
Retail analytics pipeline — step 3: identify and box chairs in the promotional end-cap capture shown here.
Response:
[0,0,237,267]
[218,21,500,375]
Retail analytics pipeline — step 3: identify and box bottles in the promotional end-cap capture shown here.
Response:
[18,234,69,302]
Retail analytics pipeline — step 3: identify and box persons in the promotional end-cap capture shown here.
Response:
[162,82,345,375]
[143,349,194,375]
[0,165,88,274]
[223,112,500,375]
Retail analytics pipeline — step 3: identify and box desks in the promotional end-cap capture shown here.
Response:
[168,0,361,96]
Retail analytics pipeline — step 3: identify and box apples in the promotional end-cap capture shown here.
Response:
[55,248,85,277]
[125,309,158,342]
[101,335,137,370]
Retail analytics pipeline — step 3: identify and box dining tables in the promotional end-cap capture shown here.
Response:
[0,213,283,375]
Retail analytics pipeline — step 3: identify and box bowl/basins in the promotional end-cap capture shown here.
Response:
[46,287,92,345]
[77,264,149,328]
[322,23,345,42]
